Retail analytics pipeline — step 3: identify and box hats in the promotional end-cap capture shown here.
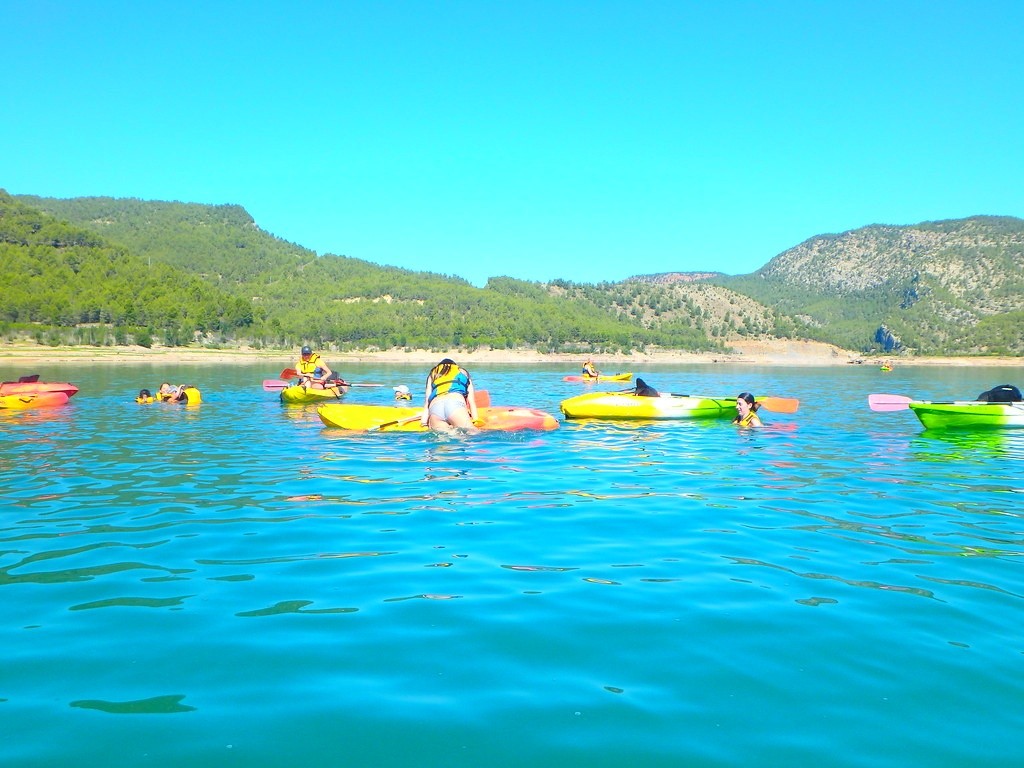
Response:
[393,385,409,394]
[302,346,311,354]
[140,389,151,398]
[738,392,754,406]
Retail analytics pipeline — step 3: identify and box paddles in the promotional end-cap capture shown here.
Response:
[595,371,601,384]
[263,379,385,392]
[869,393,1024,412]
[281,368,351,387]
[661,392,799,413]
[369,390,490,432]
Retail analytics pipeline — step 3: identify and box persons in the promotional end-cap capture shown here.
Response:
[582,358,601,377]
[733,392,761,426]
[140,382,170,398]
[393,385,413,401]
[295,346,332,389]
[421,359,478,429]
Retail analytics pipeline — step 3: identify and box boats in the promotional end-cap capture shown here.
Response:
[909,397,1024,431]
[1,373,80,411]
[561,392,762,420]
[281,378,349,402]
[562,373,632,383]
[314,402,561,433]
